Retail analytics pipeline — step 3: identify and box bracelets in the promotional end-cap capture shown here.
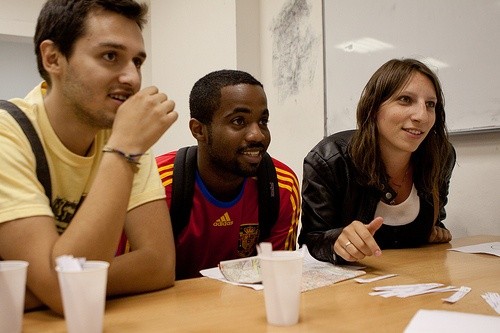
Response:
[102,145,150,173]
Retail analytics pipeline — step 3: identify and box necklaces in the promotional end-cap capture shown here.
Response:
[390,165,410,205]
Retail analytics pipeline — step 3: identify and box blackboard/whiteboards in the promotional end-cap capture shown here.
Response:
[322,0,500,139]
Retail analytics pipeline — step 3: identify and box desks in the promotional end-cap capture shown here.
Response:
[23,236,500,333]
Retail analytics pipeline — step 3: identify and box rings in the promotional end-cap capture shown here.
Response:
[344,242,351,249]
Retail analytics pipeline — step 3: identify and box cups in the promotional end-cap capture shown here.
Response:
[55,260,110,333]
[0,261,29,333]
[258,250,303,325]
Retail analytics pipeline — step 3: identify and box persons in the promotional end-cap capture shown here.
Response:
[297,58,457,265]
[115,70,301,284]
[1,0,175,318]
